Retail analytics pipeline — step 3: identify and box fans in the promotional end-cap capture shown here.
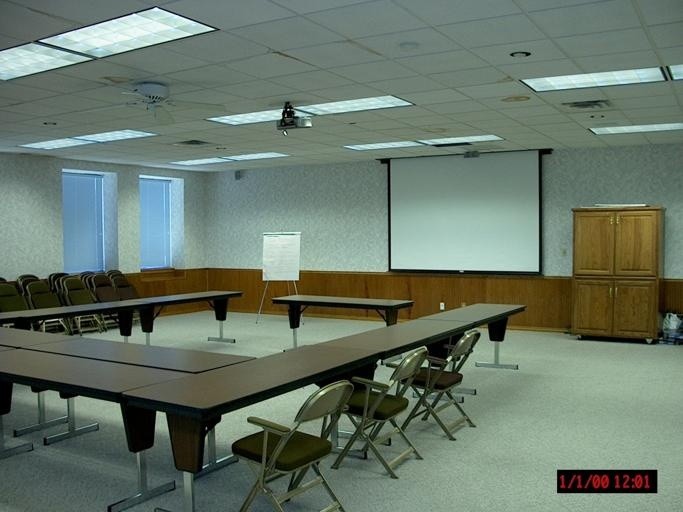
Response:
[99,74,230,125]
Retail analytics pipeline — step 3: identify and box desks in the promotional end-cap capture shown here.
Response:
[2,345,17,354]
[3,347,196,511]
[24,337,257,481]
[1,299,151,346]
[272,294,416,353]
[122,344,383,511]
[124,289,245,343]
[417,303,525,395]
[2,328,81,438]
[315,320,465,445]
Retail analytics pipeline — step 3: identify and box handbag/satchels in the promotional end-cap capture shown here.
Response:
[663,313,681,329]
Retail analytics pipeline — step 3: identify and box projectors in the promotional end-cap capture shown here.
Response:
[276,117,313,129]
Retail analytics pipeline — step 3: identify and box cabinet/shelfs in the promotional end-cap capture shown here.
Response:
[571,207,666,344]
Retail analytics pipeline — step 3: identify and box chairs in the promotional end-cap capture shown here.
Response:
[232,380,354,510]
[330,345,429,479]
[399,329,483,442]
[0,272,143,329]
